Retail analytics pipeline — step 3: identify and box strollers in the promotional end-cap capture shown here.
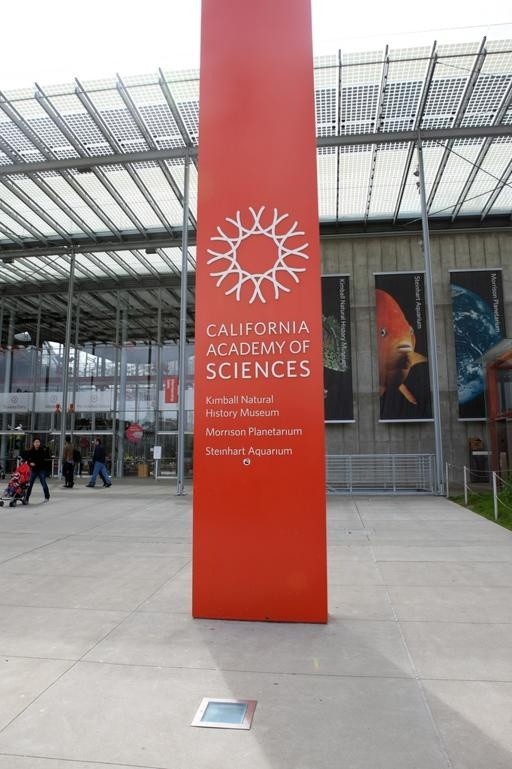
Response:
[0,462,33,507]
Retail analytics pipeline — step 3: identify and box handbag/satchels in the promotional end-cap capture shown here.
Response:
[72,449,82,463]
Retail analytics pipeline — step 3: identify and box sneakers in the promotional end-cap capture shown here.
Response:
[45,494,50,501]
[60,481,113,487]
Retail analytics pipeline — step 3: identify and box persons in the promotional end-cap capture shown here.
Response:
[61,436,76,489]
[72,444,85,476]
[20,437,51,505]
[85,436,113,488]
